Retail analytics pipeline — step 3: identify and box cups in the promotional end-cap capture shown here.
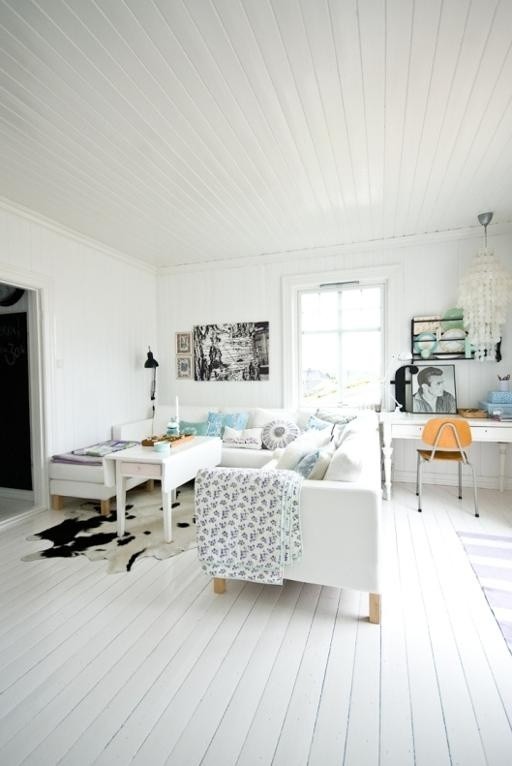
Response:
[491,408,503,421]
[421,350,430,359]
[497,381,508,391]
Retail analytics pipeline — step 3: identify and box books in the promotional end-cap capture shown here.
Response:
[84,438,139,457]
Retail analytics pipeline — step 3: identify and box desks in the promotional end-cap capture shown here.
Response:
[378,411,512,501]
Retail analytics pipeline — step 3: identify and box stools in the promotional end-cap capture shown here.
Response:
[48,440,155,518]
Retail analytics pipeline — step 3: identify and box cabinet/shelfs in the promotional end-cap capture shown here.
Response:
[410,317,503,363]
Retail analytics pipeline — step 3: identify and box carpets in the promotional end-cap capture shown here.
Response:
[18,487,197,575]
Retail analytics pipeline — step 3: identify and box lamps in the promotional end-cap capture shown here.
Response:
[144,345,159,436]
[459,211,511,362]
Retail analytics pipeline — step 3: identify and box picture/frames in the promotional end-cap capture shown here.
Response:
[174,331,192,380]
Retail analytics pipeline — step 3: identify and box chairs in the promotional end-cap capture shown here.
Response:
[413,417,479,520]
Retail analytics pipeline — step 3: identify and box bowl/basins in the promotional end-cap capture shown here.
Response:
[155,441,169,452]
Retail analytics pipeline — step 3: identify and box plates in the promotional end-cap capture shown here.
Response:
[436,355,461,359]
[439,329,466,352]
[415,333,436,353]
[441,307,465,331]
